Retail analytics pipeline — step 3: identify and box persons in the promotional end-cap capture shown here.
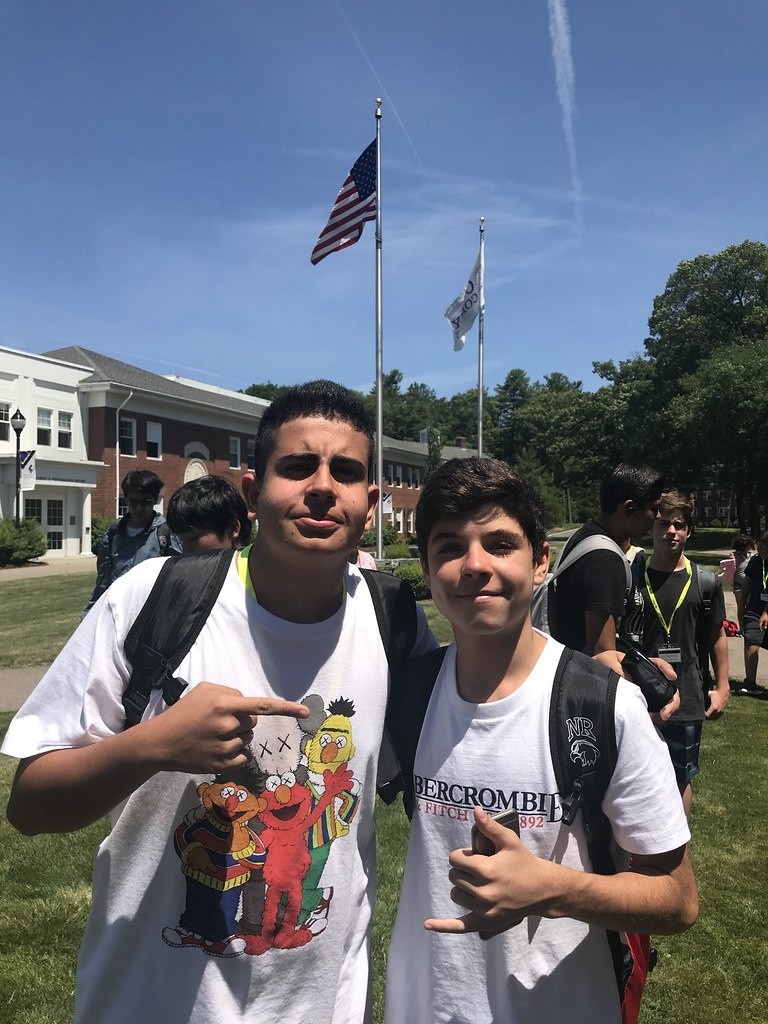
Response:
[546,461,768,818]
[7,378,681,1024]
[376,456,698,1023]
[83,468,253,617]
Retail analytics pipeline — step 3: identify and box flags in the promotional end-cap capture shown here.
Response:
[443,250,485,352]
[310,138,377,266]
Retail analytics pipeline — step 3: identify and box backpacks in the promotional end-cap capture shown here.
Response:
[530,527,632,637]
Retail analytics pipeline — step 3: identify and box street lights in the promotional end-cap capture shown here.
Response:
[9,407,27,530]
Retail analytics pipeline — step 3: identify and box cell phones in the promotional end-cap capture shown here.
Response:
[471,809,524,942]
[619,648,677,713]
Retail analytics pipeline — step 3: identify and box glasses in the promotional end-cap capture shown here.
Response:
[125,497,155,506]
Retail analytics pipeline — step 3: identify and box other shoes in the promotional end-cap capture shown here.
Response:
[741,686,763,695]
